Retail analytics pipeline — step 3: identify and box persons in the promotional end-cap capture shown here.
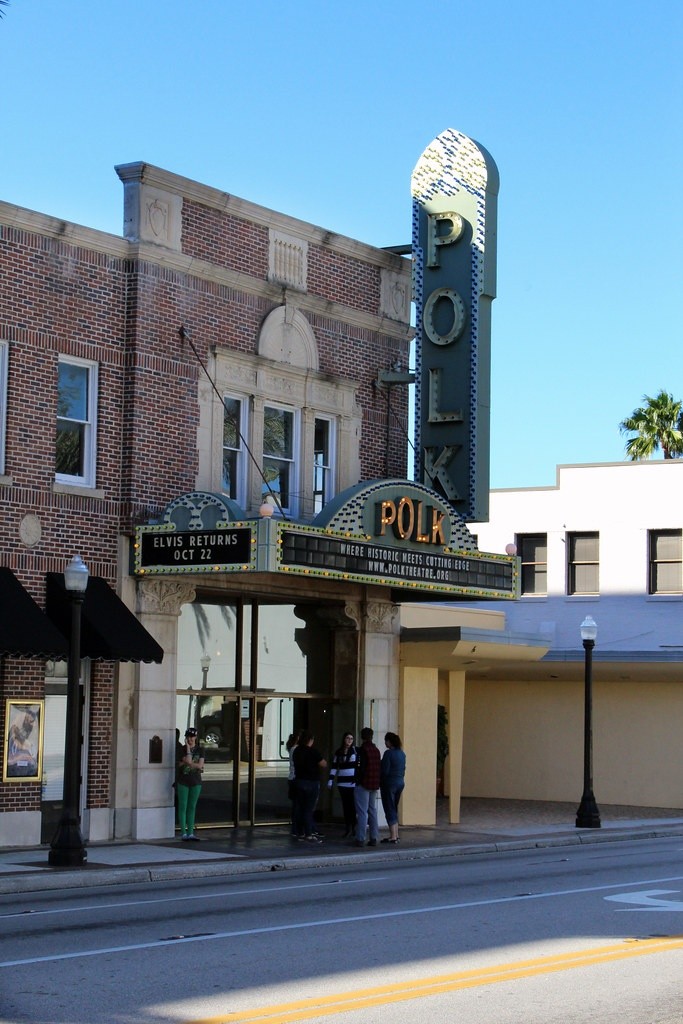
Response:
[176,728,205,840]
[381,732,406,844]
[286,729,328,843]
[328,732,357,838]
[351,727,381,846]
[9,704,39,753]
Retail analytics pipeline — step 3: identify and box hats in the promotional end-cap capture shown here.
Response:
[185,728,197,737]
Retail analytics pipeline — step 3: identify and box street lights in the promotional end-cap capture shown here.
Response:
[47,553,88,867]
[576,614,601,828]
[199,652,211,690]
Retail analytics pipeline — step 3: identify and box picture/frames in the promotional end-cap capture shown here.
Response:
[3,699,44,782]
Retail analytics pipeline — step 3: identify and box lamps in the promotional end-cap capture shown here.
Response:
[261,503,274,519]
[506,544,517,555]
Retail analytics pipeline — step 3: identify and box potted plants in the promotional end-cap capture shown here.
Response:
[436,705,448,796]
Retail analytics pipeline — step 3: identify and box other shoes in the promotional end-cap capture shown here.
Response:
[342,830,350,838]
[381,838,400,844]
[350,831,356,837]
[188,835,200,842]
[182,836,190,842]
[367,840,376,846]
[289,831,325,844]
[355,839,363,846]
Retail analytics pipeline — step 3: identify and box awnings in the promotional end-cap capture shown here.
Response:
[44,571,164,665]
[0,567,66,661]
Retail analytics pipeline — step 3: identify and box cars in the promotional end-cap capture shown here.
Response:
[200,710,235,744]
